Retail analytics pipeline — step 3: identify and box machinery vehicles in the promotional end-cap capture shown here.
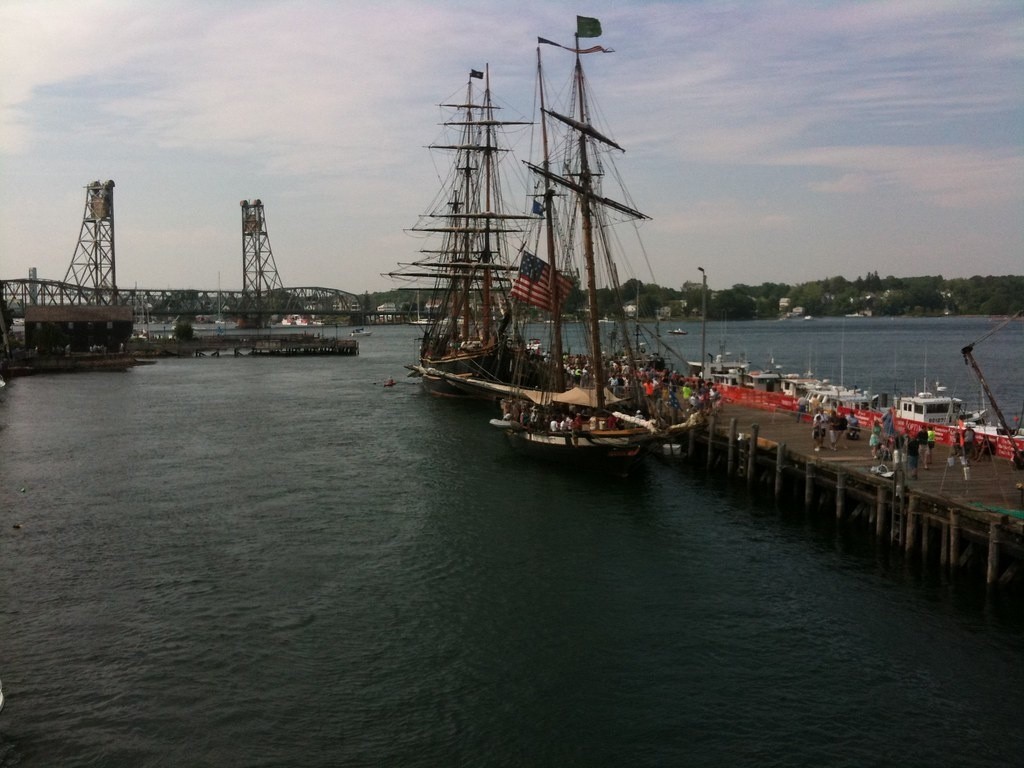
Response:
[961,310,1024,472]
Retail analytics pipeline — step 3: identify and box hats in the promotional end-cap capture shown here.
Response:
[576,413,581,417]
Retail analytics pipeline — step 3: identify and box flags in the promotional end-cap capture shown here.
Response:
[533,199,544,216]
[577,15,602,37]
[510,250,574,313]
[471,69,483,79]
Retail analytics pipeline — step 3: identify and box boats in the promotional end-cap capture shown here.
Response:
[350,329,372,336]
[710,369,1024,459]
[668,328,688,334]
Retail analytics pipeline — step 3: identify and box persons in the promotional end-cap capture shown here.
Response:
[527,341,724,423]
[119,342,124,352]
[90,344,106,353]
[868,408,935,479]
[545,407,645,431]
[50,343,72,355]
[447,334,483,350]
[796,393,861,451]
[388,376,393,385]
[953,423,1024,468]
[503,399,539,434]
[32,343,38,352]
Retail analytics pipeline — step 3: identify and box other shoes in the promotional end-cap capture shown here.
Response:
[815,448,820,451]
[925,467,929,470]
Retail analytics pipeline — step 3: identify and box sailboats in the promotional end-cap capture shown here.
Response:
[381,15,710,456]
